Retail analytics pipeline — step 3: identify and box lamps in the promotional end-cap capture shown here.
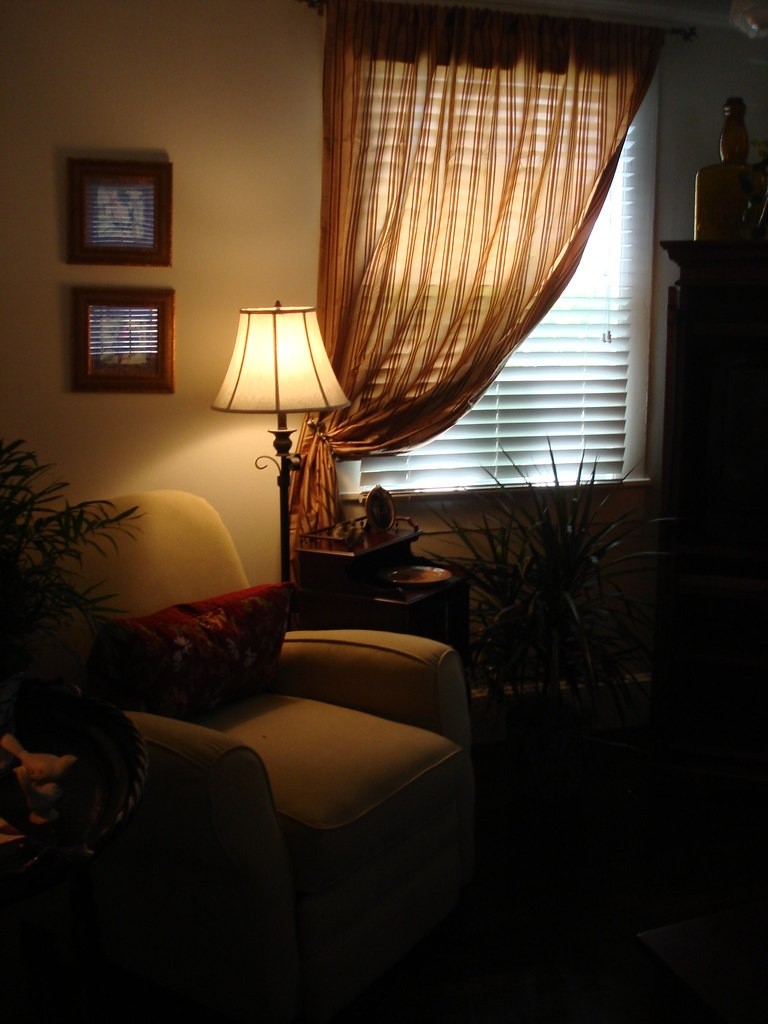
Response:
[211,300,352,582]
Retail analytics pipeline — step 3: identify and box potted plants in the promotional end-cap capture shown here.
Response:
[0,439,136,780]
[416,436,667,803]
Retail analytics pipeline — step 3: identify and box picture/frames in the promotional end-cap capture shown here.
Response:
[65,155,173,267]
[69,283,176,395]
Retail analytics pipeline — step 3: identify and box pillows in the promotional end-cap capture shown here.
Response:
[85,583,293,720]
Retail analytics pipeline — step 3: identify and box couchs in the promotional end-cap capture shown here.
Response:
[52,489,476,1024]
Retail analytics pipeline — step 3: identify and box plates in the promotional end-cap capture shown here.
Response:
[384,566,452,584]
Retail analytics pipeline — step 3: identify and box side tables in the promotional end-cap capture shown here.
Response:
[0,683,148,1024]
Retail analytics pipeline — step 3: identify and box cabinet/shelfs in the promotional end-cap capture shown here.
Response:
[644,239,768,848]
